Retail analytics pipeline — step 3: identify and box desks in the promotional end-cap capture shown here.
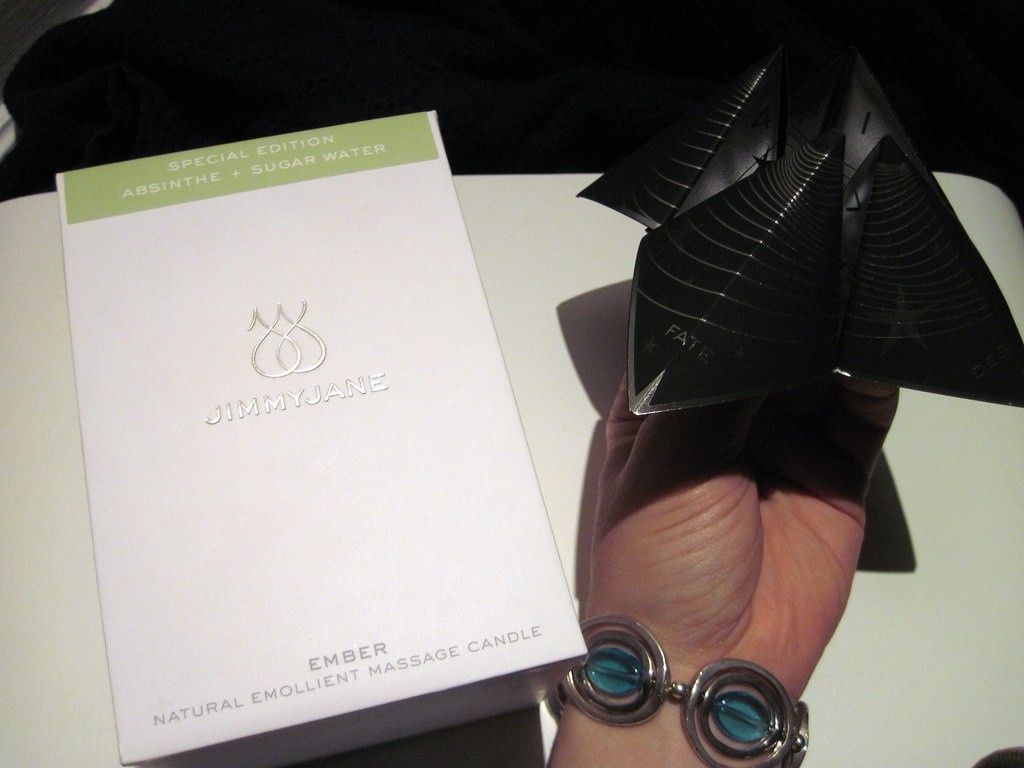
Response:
[1,167,1024,768]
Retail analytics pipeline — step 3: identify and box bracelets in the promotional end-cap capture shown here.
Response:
[559,623,810,768]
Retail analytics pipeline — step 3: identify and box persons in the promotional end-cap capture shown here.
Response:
[541,363,898,767]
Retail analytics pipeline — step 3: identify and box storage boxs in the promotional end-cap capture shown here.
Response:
[55,113,586,768]
